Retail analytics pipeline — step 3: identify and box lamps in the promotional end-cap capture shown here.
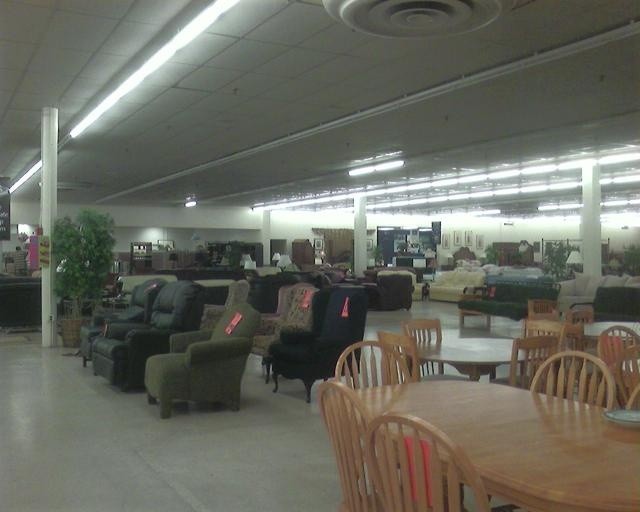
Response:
[183,194,199,207]
[566,251,583,273]
[190,233,200,241]
[321,0,518,38]
[273,252,281,267]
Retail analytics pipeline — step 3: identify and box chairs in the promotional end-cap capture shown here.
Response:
[0,272,41,333]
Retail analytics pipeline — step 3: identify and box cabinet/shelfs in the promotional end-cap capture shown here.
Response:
[206,241,262,267]
[132,242,152,274]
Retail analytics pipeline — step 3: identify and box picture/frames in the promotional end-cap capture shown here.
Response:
[367,239,373,251]
[314,238,323,249]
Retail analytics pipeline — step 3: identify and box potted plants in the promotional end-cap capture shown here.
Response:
[51,208,116,347]
